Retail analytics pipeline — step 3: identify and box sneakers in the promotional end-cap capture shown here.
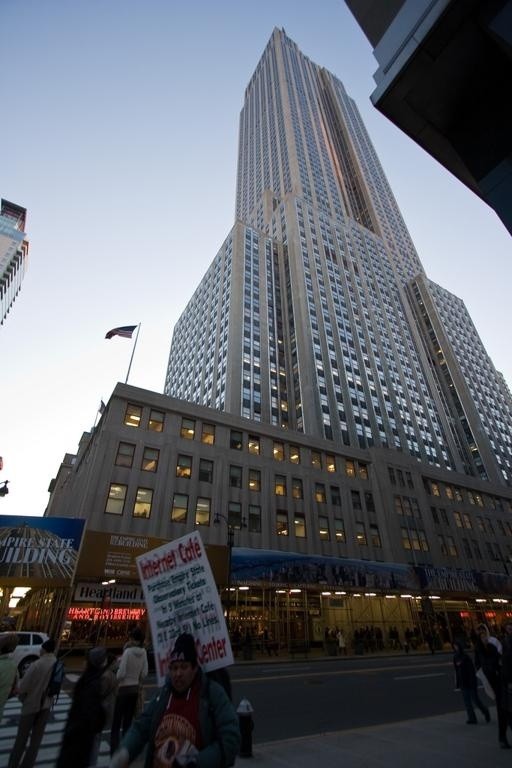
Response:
[465,708,512,749]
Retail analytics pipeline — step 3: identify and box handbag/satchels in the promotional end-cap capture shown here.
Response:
[46,661,65,698]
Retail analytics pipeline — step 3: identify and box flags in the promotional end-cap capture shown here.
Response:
[104,325,138,339]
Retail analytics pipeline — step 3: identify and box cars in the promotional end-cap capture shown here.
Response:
[0,631,50,679]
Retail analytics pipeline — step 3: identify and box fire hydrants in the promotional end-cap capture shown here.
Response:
[237,699,255,758]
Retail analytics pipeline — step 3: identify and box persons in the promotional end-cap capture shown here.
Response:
[56,646,111,768]
[110,625,149,758]
[100,653,117,730]
[0,632,21,723]
[324,617,512,750]
[8,638,65,768]
[231,626,278,660]
[106,632,243,768]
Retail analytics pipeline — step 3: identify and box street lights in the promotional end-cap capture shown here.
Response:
[213,513,234,631]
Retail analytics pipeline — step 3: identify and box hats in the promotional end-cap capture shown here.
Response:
[87,645,108,668]
[41,638,56,653]
[167,632,198,668]
